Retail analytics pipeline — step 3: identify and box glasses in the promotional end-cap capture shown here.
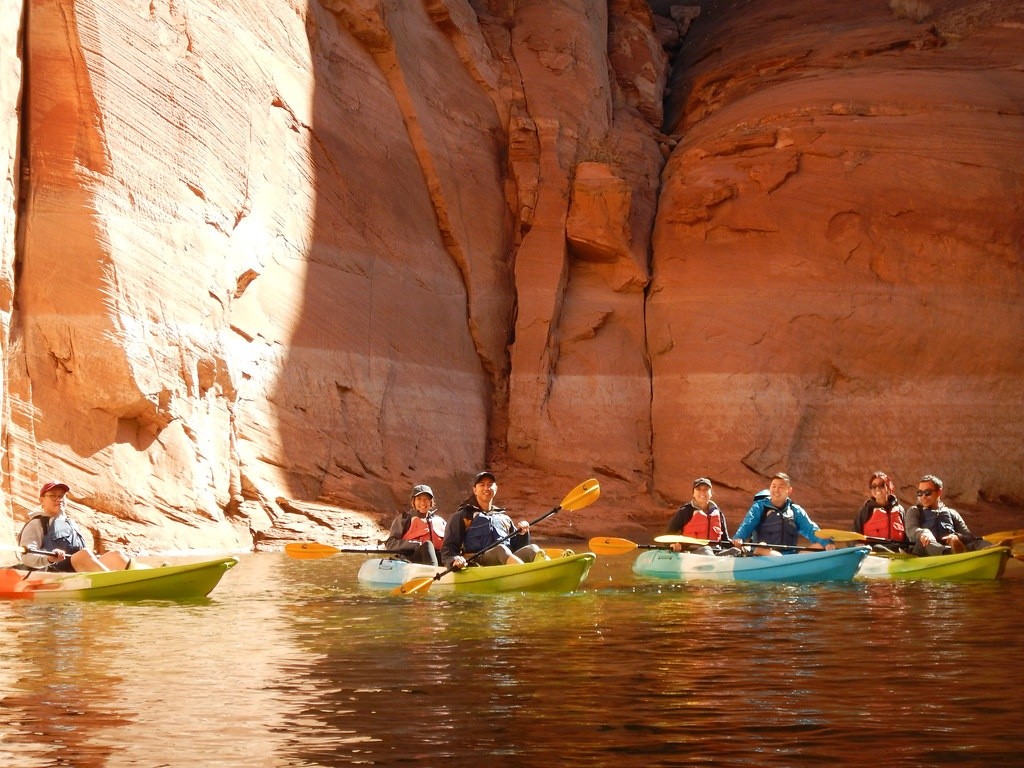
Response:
[869,482,887,490]
[42,492,65,501]
[916,489,938,497]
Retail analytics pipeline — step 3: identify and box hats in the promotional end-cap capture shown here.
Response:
[693,478,713,490]
[40,483,69,498]
[410,485,434,502]
[474,471,495,486]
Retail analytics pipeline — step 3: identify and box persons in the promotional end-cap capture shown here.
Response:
[846,472,905,553]
[440,472,575,568]
[905,476,1016,557]
[16,483,171,573]
[385,485,447,567]
[663,478,744,557]
[731,471,836,557]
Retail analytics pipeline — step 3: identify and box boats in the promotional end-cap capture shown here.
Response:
[358,549,596,599]
[851,537,1013,583]
[0,556,239,603]
[630,545,872,583]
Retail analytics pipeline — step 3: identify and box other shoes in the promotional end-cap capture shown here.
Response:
[562,549,575,558]
[160,562,170,567]
[716,547,742,557]
[532,549,545,562]
[981,537,1015,550]
[952,539,966,554]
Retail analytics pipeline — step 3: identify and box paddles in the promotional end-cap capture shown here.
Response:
[814,528,1024,563]
[284,542,564,561]
[392,477,601,595]
[653,534,826,552]
[19,545,100,559]
[588,535,722,557]
[974,529,1023,546]
